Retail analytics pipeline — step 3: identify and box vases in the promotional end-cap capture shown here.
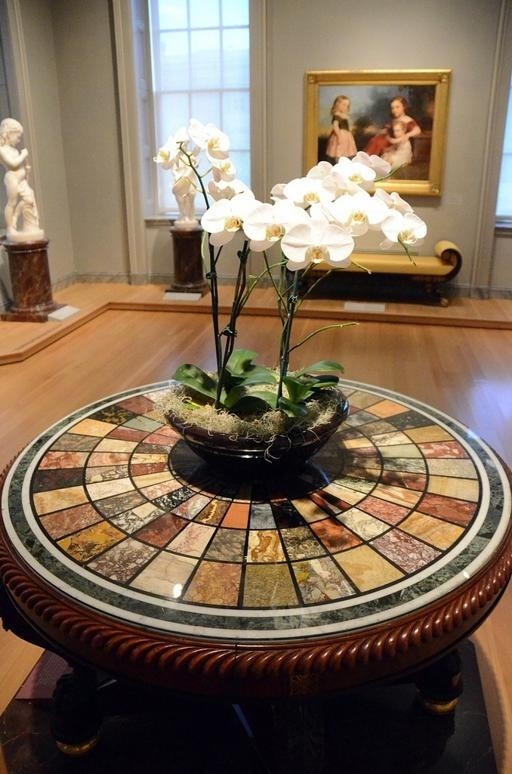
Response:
[163,387,348,477]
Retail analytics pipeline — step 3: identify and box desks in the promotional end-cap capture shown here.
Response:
[0,372,512,774]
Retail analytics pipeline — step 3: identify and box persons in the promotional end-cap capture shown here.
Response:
[363,96,422,156]
[0,116,42,237]
[326,95,357,161]
[381,121,414,170]
[160,145,200,225]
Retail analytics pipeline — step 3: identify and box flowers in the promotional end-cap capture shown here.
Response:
[154,119,427,433]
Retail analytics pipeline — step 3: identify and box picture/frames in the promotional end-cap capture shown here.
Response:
[303,69,452,197]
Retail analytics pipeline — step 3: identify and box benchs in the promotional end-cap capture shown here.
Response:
[281,240,463,307]
